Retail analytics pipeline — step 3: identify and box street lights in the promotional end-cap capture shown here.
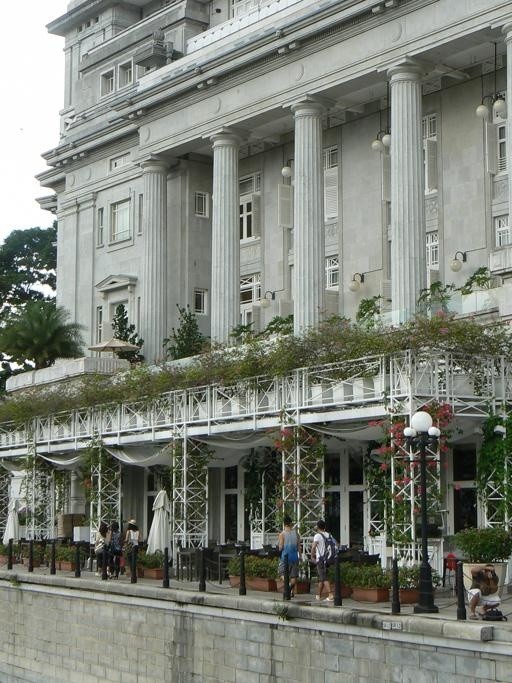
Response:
[402,410,444,614]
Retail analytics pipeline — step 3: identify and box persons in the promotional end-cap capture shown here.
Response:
[124,518,140,575]
[277,514,303,599]
[466,562,501,620]
[111,521,124,579]
[94,522,111,577]
[310,519,339,603]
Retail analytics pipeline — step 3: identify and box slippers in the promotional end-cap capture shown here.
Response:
[316,595,334,602]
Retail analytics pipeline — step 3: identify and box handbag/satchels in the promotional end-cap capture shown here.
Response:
[94,538,106,554]
[485,611,502,621]
[123,543,132,553]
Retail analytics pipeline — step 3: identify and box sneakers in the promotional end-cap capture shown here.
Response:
[470,615,478,620]
[288,583,294,597]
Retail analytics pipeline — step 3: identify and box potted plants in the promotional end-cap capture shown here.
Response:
[274,558,312,594]
[47,545,85,572]
[347,564,389,603]
[328,563,353,599]
[228,555,277,591]
[387,564,442,603]
[448,528,511,599]
[1,541,47,569]
[136,550,169,580]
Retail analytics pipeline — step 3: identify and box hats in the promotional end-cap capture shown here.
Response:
[128,520,137,526]
[284,518,292,526]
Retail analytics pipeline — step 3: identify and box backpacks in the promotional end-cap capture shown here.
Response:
[319,533,338,567]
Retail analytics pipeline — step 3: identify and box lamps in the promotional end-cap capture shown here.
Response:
[372,82,391,151]
[476,43,507,119]
[281,159,294,177]
[348,272,364,291]
[450,250,467,272]
[260,290,275,308]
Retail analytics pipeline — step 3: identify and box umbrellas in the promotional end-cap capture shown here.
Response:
[145,488,172,570]
[3,497,23,559]
